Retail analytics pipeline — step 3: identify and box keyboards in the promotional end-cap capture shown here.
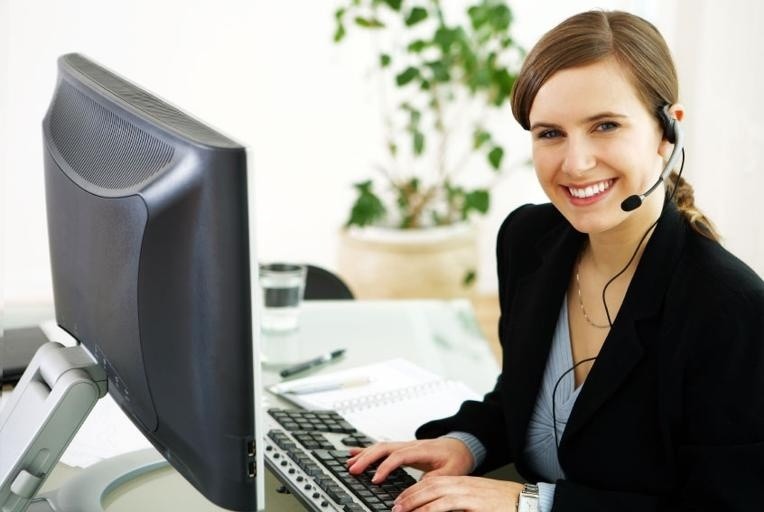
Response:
[266,409,417,512]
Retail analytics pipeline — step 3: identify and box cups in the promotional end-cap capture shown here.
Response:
[258,264,306,339]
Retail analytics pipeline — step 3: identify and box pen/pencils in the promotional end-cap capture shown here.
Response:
[281,348,346,378]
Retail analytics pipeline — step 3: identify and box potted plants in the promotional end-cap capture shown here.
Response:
[332,0,529,301]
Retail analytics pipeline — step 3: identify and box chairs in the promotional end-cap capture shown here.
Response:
[260,263,355,301]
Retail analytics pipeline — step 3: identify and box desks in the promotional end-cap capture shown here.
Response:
[0,298,502,512]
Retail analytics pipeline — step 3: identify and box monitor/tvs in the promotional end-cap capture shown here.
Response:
[0,53,266,512]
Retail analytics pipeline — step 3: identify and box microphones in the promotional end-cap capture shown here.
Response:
[621,104,685,212]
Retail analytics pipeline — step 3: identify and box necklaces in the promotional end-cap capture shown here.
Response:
[576,258,613,328]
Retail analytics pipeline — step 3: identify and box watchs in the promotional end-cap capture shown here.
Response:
[515,482,539,512]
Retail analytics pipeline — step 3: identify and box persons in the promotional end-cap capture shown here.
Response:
[343,8,764,511]
